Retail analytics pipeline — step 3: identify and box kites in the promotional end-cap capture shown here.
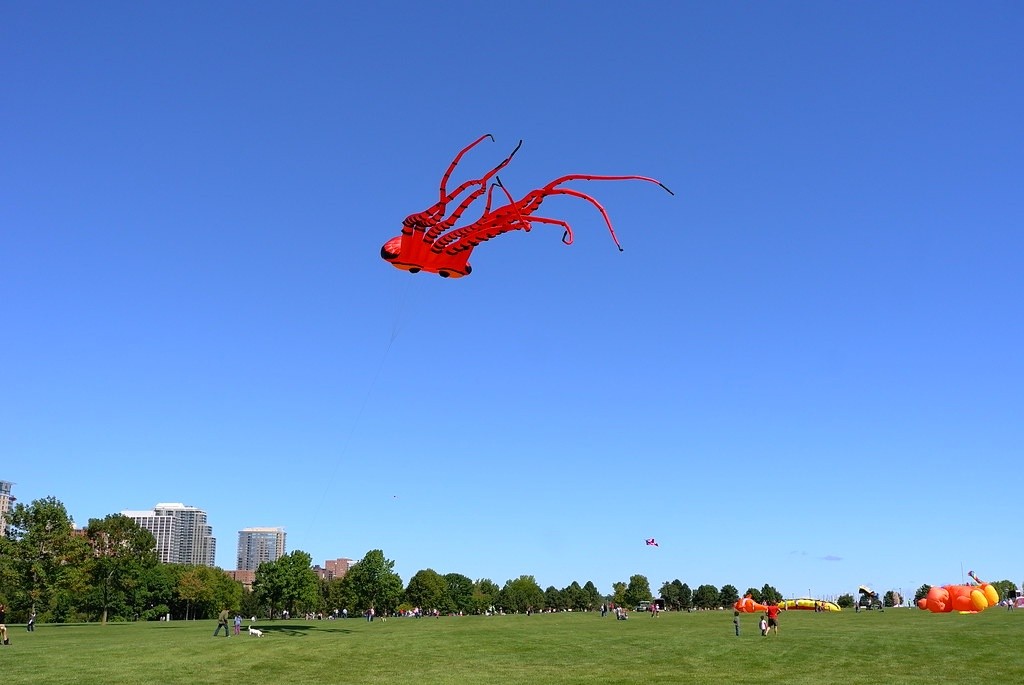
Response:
[381,132,674,279]
[646,539,658,546]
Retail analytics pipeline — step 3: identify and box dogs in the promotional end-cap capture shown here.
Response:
[248,625,264,638]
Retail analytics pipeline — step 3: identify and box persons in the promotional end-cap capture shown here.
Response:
[650,603,660,617]
[877,601,882,609]
[1000,598,1014,612]
[908,598,919,609]
[601,602,629,620]
[733,611,740,636]
[795,598,799,609]
[784,599,788,611]
[813,601,831,612]
[234,611,242,636]
[664,604,724,613]
[476,603,587,616]
[383,606,464,619]
[213,607,230,637]
[756,597,784,636]
[0,603,9,645]
[855,601,860,613]
[134,612,138,622]
[362,606,375,622]
[250,607,348,622]
[27,611,36,632]
[160,616,165,621]
